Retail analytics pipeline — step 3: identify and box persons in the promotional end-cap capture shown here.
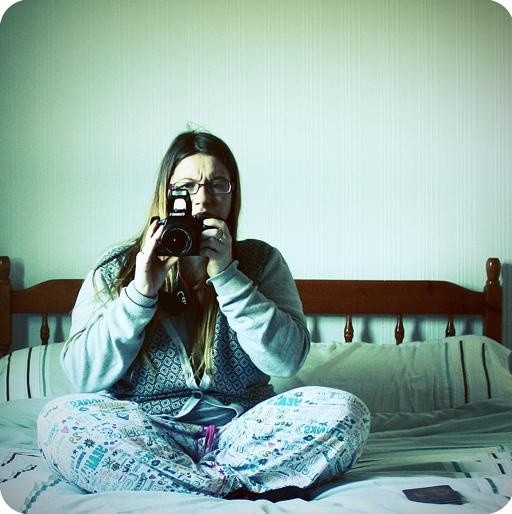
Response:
[38,131,372,498]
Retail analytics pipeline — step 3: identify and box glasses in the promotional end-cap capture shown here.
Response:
[166,178,236,197]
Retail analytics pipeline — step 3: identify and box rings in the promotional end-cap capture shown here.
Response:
[218,233,228,243]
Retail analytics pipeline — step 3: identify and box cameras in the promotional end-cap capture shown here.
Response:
[152,189,218,256]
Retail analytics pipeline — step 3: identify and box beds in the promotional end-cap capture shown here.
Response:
[1,250,509,514]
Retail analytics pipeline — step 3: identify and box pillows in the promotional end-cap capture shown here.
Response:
[267,332,511,417]
[2,335,88,399]
